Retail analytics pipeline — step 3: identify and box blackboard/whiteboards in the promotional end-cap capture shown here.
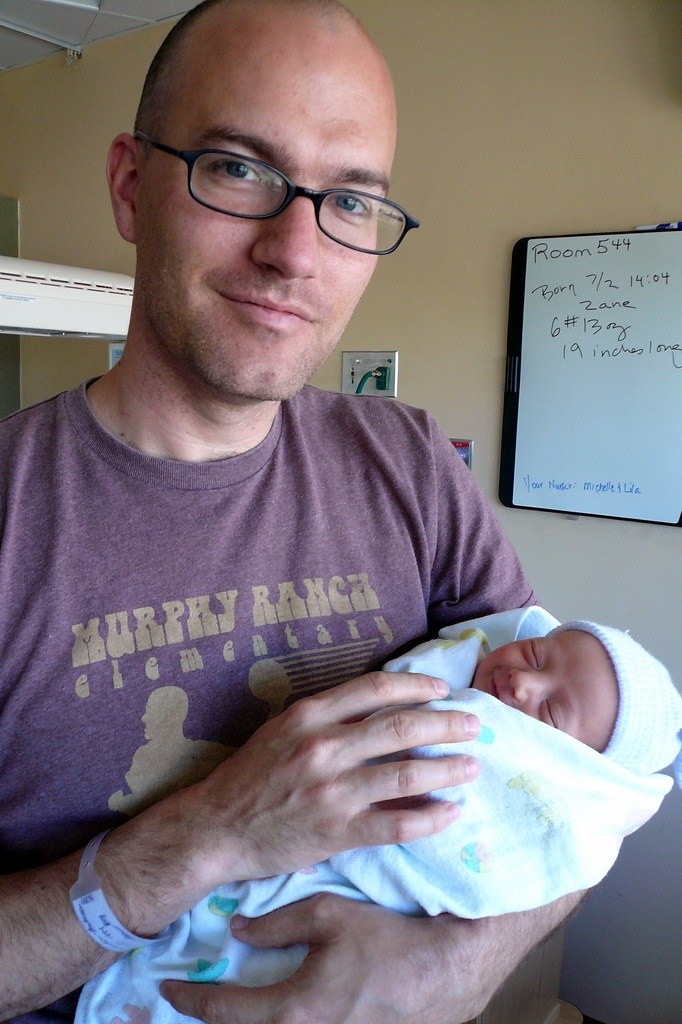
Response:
[498,228,682,527]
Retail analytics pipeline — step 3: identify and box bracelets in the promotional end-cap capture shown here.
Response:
[70,829,183,955]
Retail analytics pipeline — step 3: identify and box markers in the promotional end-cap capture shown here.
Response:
[634,221,682,230]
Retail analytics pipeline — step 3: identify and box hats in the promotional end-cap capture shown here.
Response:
[545,618,681,773]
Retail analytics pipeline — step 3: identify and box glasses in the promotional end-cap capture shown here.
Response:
[134,130,420,255]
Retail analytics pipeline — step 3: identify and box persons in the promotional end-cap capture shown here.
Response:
[72,614,680,1023]
[0,2,593,1023]
